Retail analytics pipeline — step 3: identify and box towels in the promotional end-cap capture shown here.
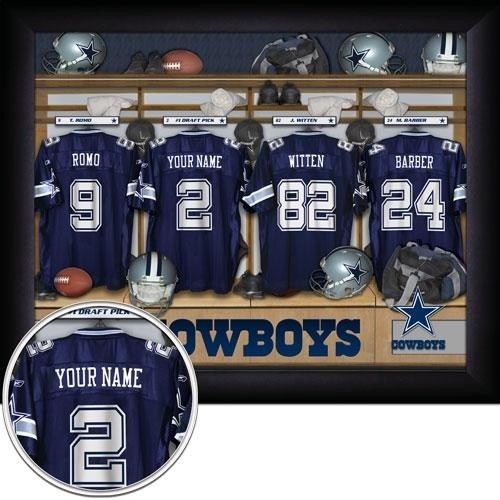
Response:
[82,88,409,125]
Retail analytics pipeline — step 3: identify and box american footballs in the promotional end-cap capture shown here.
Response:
[54,267,93,298]
[161,50,203,74]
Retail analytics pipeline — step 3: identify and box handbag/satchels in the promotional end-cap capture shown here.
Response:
[381,242,467,308]
[249,34,330,75]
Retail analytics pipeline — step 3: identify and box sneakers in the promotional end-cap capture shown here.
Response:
[123,51,165,73]
[232,271,263,298]
[258,80,301,105]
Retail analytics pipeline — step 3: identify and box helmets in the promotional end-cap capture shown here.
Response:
[53,31,106,73]
[339,32,396,75]
[421,32,466,74]
[126,254,178,307]
[320,246,373,299]
